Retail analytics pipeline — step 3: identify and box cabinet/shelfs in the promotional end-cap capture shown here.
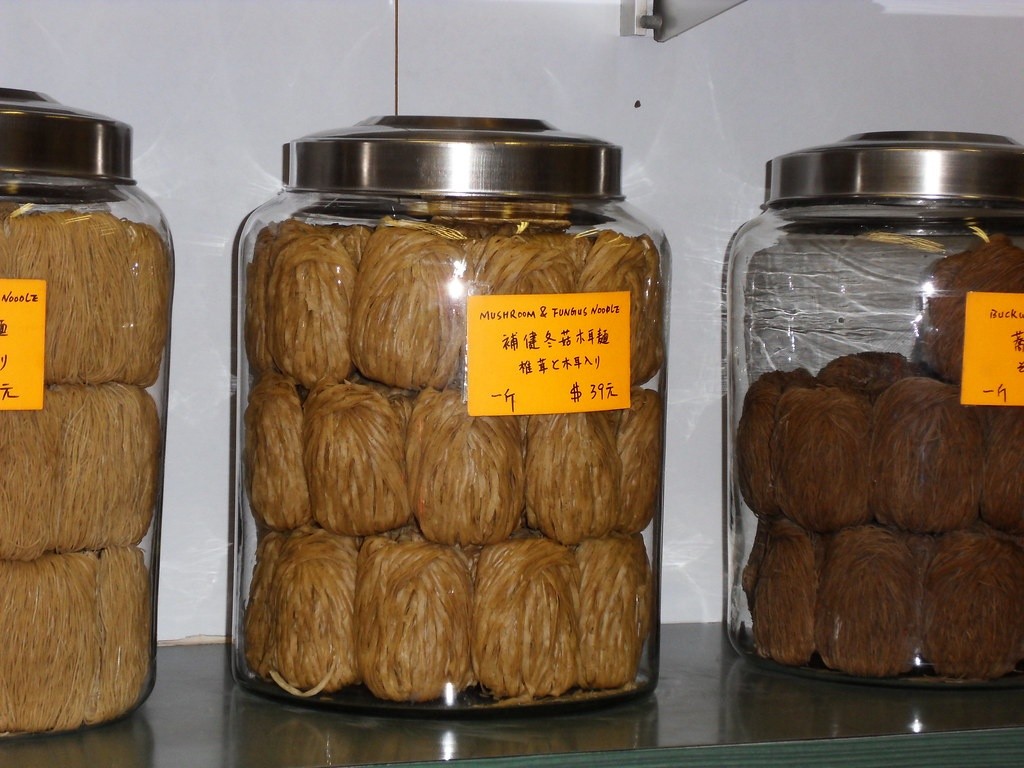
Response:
[1,623,1024,768]
